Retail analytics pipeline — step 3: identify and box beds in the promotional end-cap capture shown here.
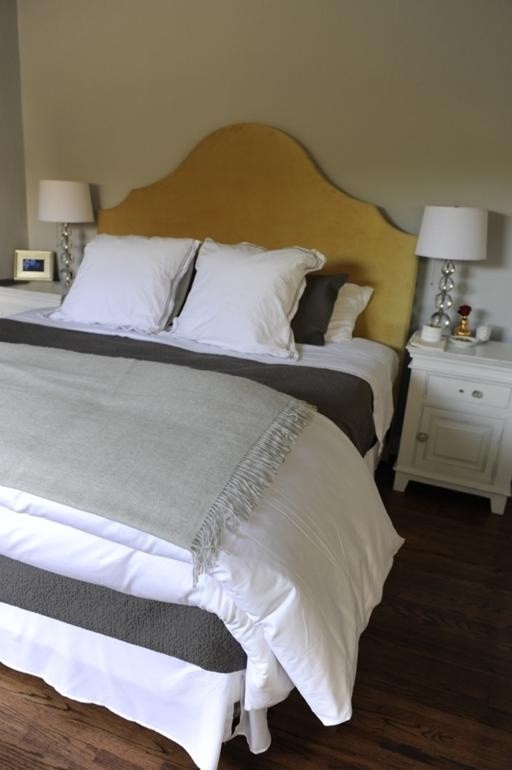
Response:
[0,120,423,770]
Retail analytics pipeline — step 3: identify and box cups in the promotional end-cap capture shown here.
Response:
[421,323,443,343]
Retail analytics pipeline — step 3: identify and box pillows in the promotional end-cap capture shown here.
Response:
[287,272,348,346]
[323,282,374,346]
[49,232,200,335]
[170,235,328,363]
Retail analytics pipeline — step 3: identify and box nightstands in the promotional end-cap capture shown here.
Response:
[0,281,69,318]
[391,328,512,516]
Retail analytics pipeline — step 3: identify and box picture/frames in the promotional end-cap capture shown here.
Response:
[12,248,55,282]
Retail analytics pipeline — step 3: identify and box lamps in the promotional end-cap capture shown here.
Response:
[414,203,488,339]
[37,180,96,288]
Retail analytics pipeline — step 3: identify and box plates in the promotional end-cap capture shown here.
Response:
[449,334,479,349]
